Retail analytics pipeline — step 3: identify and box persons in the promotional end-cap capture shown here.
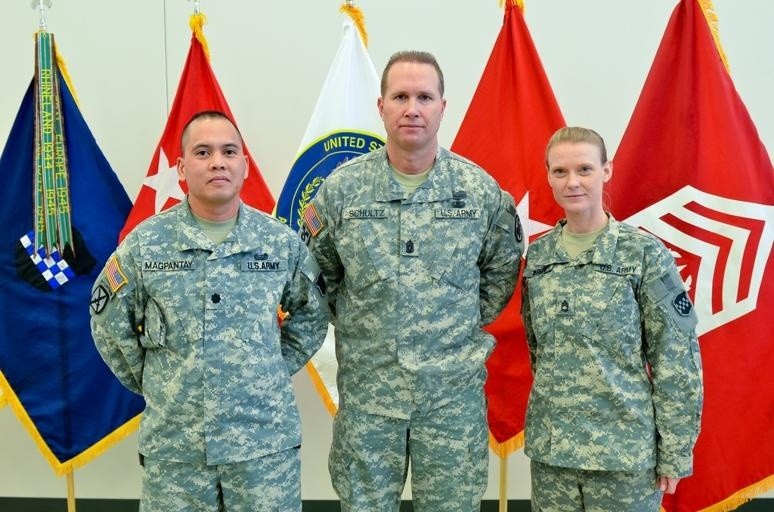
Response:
[90,110,335,512]
[519,127,705,511]
[295,47,525,512]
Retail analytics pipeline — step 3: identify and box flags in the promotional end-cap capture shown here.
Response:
[0,29,146,477]
[446,0,571,459]
[603,0,774,512]
[117,13,287,331]
[269,3,390,418]
